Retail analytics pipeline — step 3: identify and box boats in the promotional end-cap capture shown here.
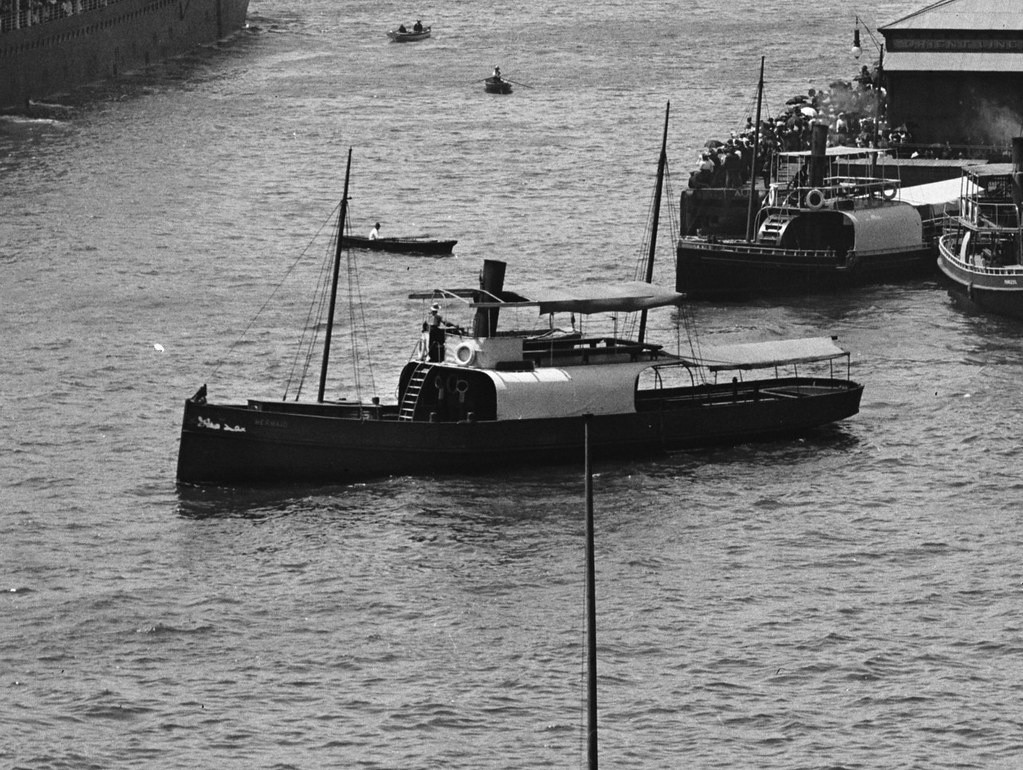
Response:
[175,148,865,487]
[386,24,432,42]
[673,49,982,293]
[0,0,250,110]
[483,77,513,94]
[934,135,1023,315]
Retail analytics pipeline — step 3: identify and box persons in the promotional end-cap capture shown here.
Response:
[493,65,501,79]
[369,222,384,240]
[419,302,455,361]
[399,24,406,33]
[686,65,1012,189]
[414,19,423,33]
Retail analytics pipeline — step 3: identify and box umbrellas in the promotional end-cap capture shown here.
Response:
[800,107,818,117]
[794,95,808,100]
[785,98,801,105]
[704,139,724,150]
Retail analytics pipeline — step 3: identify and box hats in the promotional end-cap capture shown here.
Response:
[431,302,441,311]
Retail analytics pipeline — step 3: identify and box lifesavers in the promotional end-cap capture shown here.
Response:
[455,379,469,393]
[806,189,824,211]
[881,183,898,199]
[453,342,476,367]
